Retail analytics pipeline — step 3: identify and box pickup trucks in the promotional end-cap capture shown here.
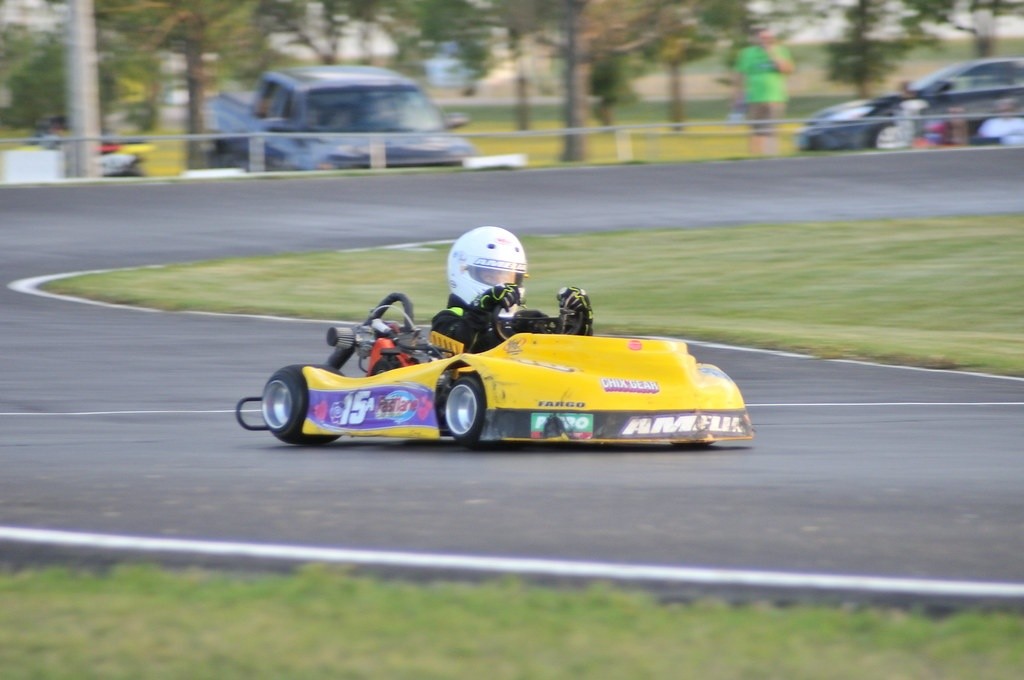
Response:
[204,65,470,172]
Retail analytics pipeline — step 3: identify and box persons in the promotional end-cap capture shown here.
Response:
[730,19,795,155]
[430,226,594,354]
[897,81,1024,146]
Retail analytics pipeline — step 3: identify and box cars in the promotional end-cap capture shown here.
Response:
[789,57,1023,152]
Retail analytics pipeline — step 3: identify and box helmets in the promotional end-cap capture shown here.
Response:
[447,226,528,319]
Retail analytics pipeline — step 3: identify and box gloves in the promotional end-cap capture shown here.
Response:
[556,285,594,336]
[462,282,521,331]
